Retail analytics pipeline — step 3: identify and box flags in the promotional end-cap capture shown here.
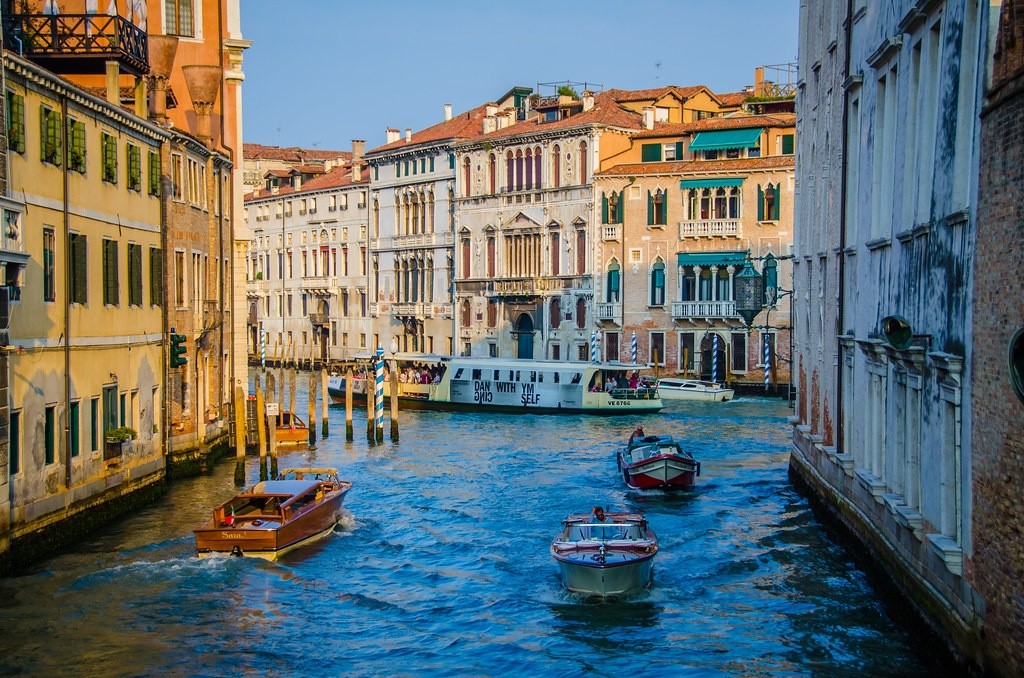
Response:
[225,505,235,525]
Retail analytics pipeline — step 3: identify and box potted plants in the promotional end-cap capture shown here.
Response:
[557,86,573,104]
[106,426,137,444]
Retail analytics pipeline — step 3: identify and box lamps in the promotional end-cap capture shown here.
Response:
[730,248,795,410]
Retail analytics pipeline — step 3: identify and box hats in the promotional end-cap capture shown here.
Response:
[637,426,643,429]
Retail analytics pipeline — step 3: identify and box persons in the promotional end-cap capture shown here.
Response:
[591,372,651,399]
[628,426,645,456]
[589,506,621,539]
[353,361,447,397]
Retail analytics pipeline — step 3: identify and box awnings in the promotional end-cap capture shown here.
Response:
[676,250,748,266]
[688,127,764,152]
[679,177,748,190]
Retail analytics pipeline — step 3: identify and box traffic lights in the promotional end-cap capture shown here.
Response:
[170,333,187,368]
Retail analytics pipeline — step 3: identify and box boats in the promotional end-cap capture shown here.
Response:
[192,468,352,564]
[653,378,735,403]
[326,352,668,415]
[550,512,660,596]
[617,436,701,489]
